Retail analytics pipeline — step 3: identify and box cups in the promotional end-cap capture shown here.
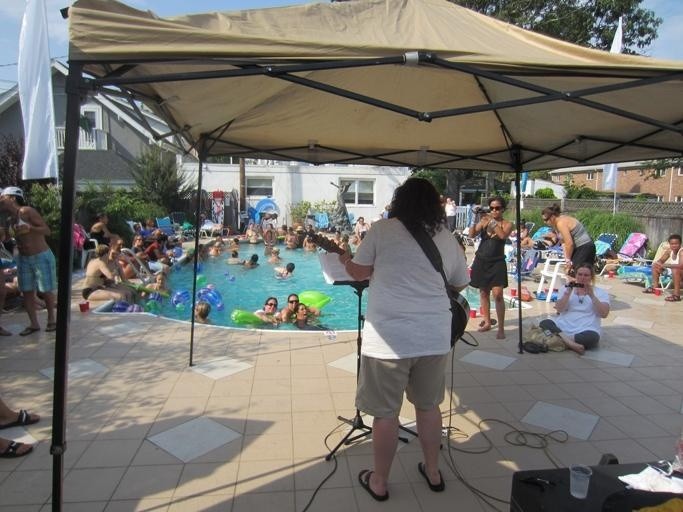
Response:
[570,463,592,499]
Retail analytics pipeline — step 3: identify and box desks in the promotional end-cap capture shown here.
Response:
[510,454,683,512]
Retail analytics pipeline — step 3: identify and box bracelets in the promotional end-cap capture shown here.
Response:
[488,219,497,229]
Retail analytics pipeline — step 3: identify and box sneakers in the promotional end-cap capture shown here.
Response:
[537,341,548,353]
[519,340,538,354]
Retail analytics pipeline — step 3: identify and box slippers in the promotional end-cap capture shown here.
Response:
[358,469,389,501]
[0,437,32,458]
[45,323,56,331]
[19,328,40,336]
[418,462,445,491]
[0,409,39,430]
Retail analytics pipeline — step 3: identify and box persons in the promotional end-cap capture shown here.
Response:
[146,219,173,265]
[131,234,169,273]
[277,225,287,236]
[134,224,162,257]
[0,226,46,310]
[245,223,259,245]
[334,231,341,245]
[214,236,222,248]
[256,225,267,235]
[354,216,371,245]
[642,235,683,301]
[228,238,240,250]
[284,227,299,249]
[279,293,321,323]
[380,205,390,220]
[444,198,457,232]
[111,234,147,277]
[89,212,119,245]
[0,261,13,337]
[194,302,211,324]
[140,272,168,297]
[303,230,317,252]
[274,263,295,279]
[337,179,472,501]
[295,225,305,248]
[512,230,557,250]
[539,263,610,355]
[0,186,57,336]
[81,245,131,305]
[306,225,312,232]
[263,223,276,255]
[339,234,349,250]
[107,243,137,295]
[245,254,259,268]
[268,247,283,263]
[227,251,244,265]
[254,297,280,324]
[293,304,308,320]
[469,196,513,339]
[193,243,205,261]
[209,246,220,257]
[541,203,596,281]
[504,219,528,263]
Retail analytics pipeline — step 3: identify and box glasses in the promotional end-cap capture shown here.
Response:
[290,300,299,303]
[266,303,277,307]
[490,206,503,210]
[544,213,552,221]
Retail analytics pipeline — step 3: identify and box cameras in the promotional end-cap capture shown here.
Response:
[473,204,492,223]
[564,280,584,289]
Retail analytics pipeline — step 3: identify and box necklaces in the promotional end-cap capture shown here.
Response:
[576,287,586,304]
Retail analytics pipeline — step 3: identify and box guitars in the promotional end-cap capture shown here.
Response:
[297,229,470,348]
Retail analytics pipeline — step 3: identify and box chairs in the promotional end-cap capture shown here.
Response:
[201,219,229,237]
[617,242,673,292]
[537,257,572,303]
[532,226,551,241]
[171,212,208,238]
[525,222,535,233]
[126,220,154,249]
[594,233,618,257]
[73,224,153,286]
[155,216,176,236]
[596,232,648,276]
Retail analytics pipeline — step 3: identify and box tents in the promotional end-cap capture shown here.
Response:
[50,0,683,512]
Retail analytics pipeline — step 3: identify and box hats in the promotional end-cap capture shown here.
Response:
[1,186,25,205]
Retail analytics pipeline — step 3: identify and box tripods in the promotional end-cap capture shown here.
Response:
[318,249,409,461]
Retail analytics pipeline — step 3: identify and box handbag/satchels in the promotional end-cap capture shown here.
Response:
[524,324,568,352]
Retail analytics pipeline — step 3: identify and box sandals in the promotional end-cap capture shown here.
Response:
[665,294,681,301]
[643,286,655,293]
